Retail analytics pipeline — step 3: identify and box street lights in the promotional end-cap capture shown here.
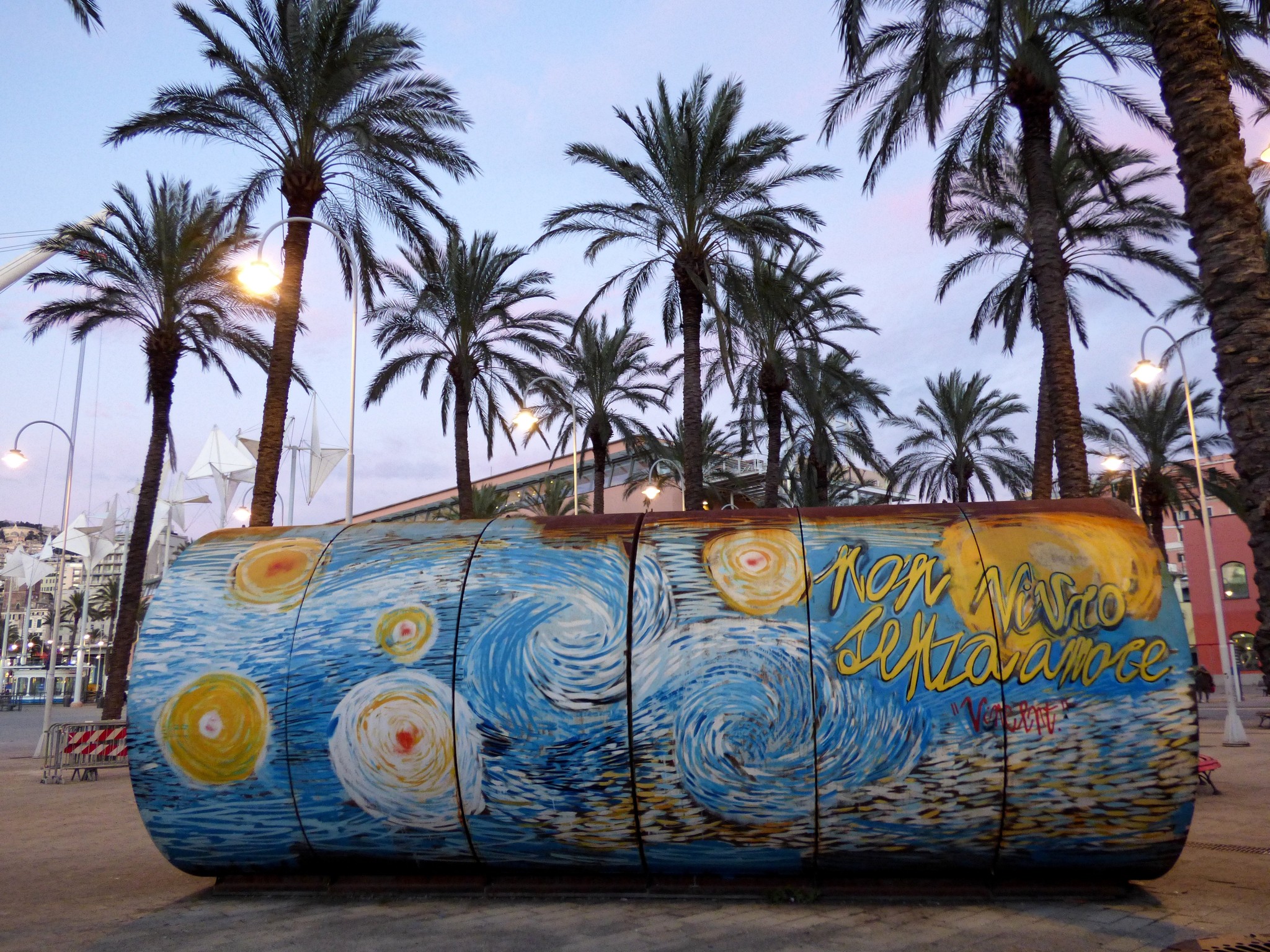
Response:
[244,215,358,521]
[96,631,104,693]
[1133,323,1252,745]
[3,420,73,759]
[1108,430,1147,521]
[85,633,92,665]
[28,635,45,657]
[508,376,582,516]
[645,457,685,510]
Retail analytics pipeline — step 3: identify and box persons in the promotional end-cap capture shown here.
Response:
[97,686,103,698]
[5,689,9,699]
[9,659,13,666]
[1195,664,1205,704]
[123,691,127,706]
[1200,667,1216,703]
[64,682,67,688]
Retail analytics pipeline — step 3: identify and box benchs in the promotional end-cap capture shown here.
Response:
[1196,752,1224,796]
